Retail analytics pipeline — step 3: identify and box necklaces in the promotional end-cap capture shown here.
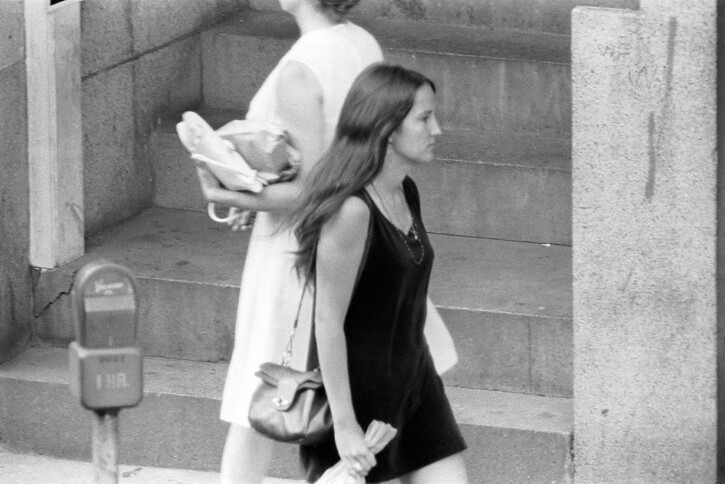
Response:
[373,181,425,265]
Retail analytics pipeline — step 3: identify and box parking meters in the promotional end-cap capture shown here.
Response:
[66,257,145,484]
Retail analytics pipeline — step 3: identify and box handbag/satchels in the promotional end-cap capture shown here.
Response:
[176,110,301,196]
[248,362,334,444]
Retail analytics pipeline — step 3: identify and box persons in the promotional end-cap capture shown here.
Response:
[197,0,458,484]
[272,62,468,484]
